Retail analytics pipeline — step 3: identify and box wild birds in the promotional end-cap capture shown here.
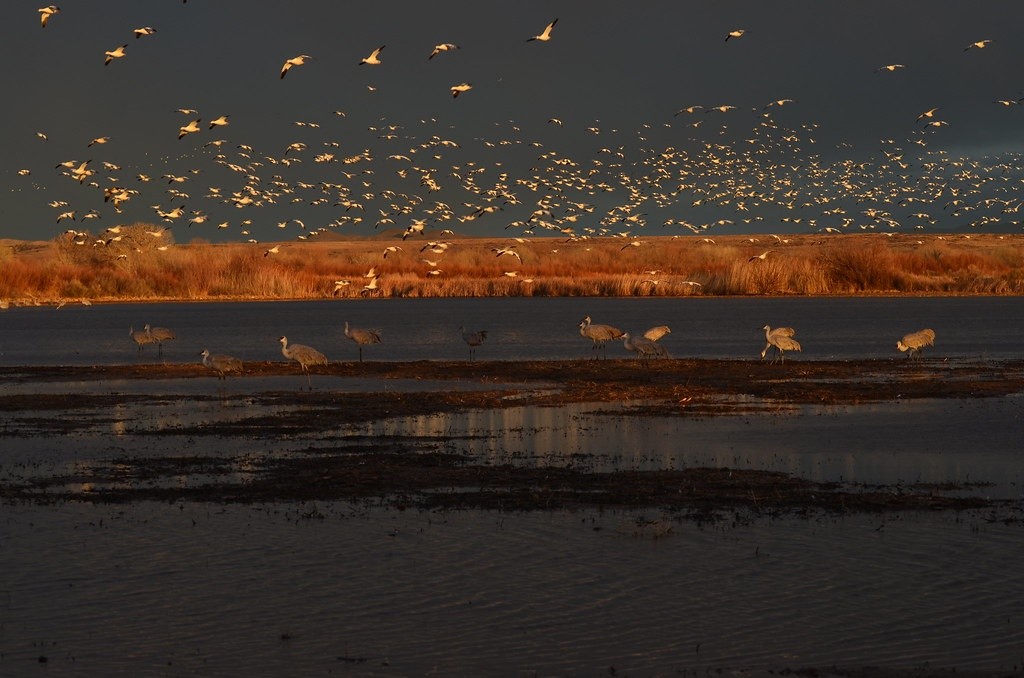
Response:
[10,6,1023,296]
[459,326,487,361]
[759,325,801,366]
[199,350,243,379]
[579,316,674,370]
[129,324,176,357]
[345,320,383,362]
[896,328,935,369]
[278,336,327,390]
